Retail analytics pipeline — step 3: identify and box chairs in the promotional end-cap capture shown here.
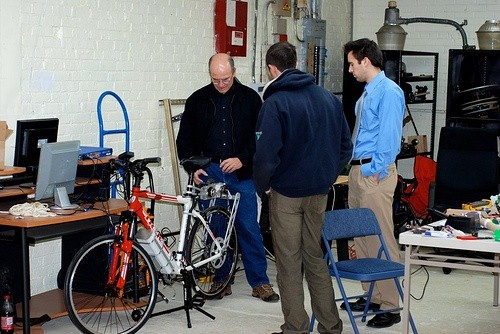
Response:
[310,208,418,334]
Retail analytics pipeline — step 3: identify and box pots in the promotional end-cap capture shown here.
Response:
[475,20,500,50]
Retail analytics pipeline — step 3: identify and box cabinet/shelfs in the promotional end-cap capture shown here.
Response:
[0,155,151,334]
[343,52,438,221]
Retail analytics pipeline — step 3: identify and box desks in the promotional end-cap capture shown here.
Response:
[399,219,500,334]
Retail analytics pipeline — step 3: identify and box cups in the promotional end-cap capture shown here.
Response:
[494,228,500,242]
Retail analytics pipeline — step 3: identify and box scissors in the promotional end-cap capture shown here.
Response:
[457,236,493,240]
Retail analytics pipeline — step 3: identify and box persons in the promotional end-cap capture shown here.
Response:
[252,40,354,334]
[176,52,279,302]
[340,37,405,329]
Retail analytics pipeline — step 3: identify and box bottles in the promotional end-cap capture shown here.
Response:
[0,296,15,334]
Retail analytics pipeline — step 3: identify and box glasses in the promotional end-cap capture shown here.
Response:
[210,71,233,86]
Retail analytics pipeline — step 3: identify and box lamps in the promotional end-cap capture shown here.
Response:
[475,20,500,50]
[375,1,468,50]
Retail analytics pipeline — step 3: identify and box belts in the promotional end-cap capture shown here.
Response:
[350,158,372,165]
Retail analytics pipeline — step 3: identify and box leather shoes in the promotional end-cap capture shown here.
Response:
[340,296,381,312]
[366,310,401,328]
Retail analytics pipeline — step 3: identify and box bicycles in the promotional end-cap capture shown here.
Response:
[64,152,241,334]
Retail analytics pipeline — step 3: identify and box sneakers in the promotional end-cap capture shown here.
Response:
[252,283,279,302]
[206,283,232,301]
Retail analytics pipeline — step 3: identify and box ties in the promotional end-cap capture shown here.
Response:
[346,90,366,169]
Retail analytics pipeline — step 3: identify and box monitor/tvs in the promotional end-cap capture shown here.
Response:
[12,118,60,189]
[34,139,80,210]
